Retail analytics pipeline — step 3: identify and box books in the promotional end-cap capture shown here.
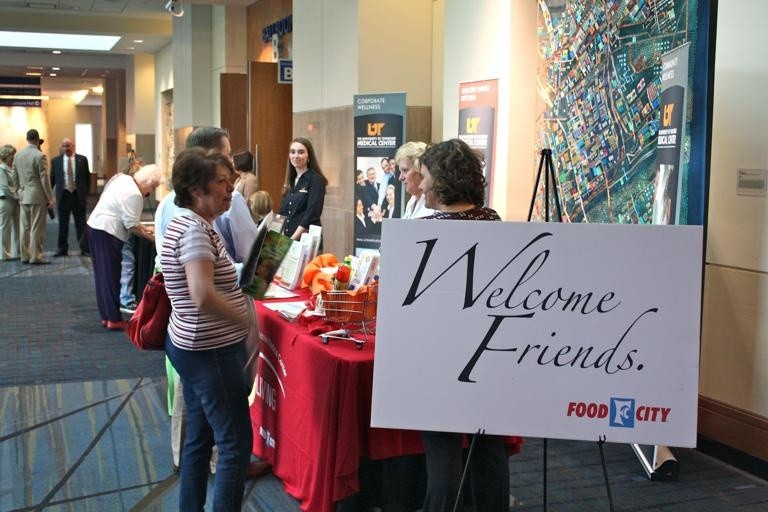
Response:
[238,224,294,301]
[270,223,323,293]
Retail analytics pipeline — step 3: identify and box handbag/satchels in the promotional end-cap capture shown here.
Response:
[124,273,171,350]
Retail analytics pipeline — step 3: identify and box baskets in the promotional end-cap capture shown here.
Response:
[318,286,378,324]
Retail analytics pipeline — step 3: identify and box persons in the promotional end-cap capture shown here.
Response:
[230,148,258,201]
[0,145,20,262]
[394,141,438,219]
[49,137,93,257]
[11,128,57,265]
[155,125,276,481]
[34,138,45,153]
[160,145,254,511]
[415,137,512,510]
[244,189,273,227]
[354,156,396,241]
[100,157,155,314]
[278,137,327,256]
[85,163,162,331]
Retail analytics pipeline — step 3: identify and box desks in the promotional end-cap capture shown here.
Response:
[247,279,524,512]
[133,224,157,302]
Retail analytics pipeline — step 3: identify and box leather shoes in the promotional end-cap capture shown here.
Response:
[101,319,129,331]
[245,461,271,479]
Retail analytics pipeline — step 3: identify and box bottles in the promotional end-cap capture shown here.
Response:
[47,202,56,220]
[333,255,353,289]
[370,275,379,285]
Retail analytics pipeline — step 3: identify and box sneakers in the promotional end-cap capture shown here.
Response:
[120,301,139,313]
